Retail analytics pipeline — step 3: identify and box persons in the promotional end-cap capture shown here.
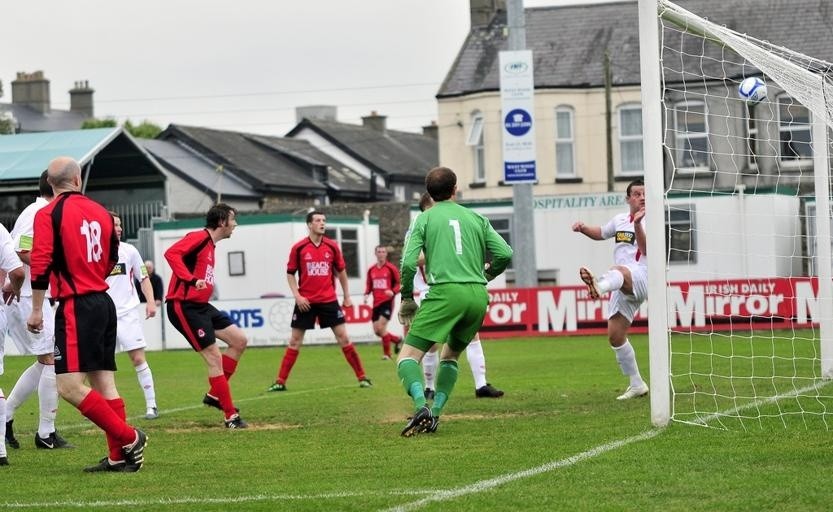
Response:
[397,167,513,439]
[105,211,159,421]
[0,220,26,467]
[266,211,372,392]
[362,245,404,362]
[27,155,148,476]
[403,194,504,400]
[136,257,164,307]
[5,170,70,451]
[572,178,651,401]
[165,204,249,432]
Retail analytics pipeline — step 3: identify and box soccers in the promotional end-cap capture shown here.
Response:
[737,78,768,106]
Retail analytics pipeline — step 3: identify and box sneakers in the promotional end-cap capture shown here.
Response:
[427,416,439,433]
[35,429,75,450]
[224,414,248,429]
[579,267,602,300]
[202,394,240,414]
[6,420,20,449]
[122,427,149,473]
[424,388,435,401]
[400,404,433,436]
[384,355,391,360]
[395,336,404,354]
[82,460,125,473]
[268,384,287,392]
[0,457,9,466]
[145,407,158,419]
[360,378,373,387]
[475,383,504,397]
[616,382,649,400]
